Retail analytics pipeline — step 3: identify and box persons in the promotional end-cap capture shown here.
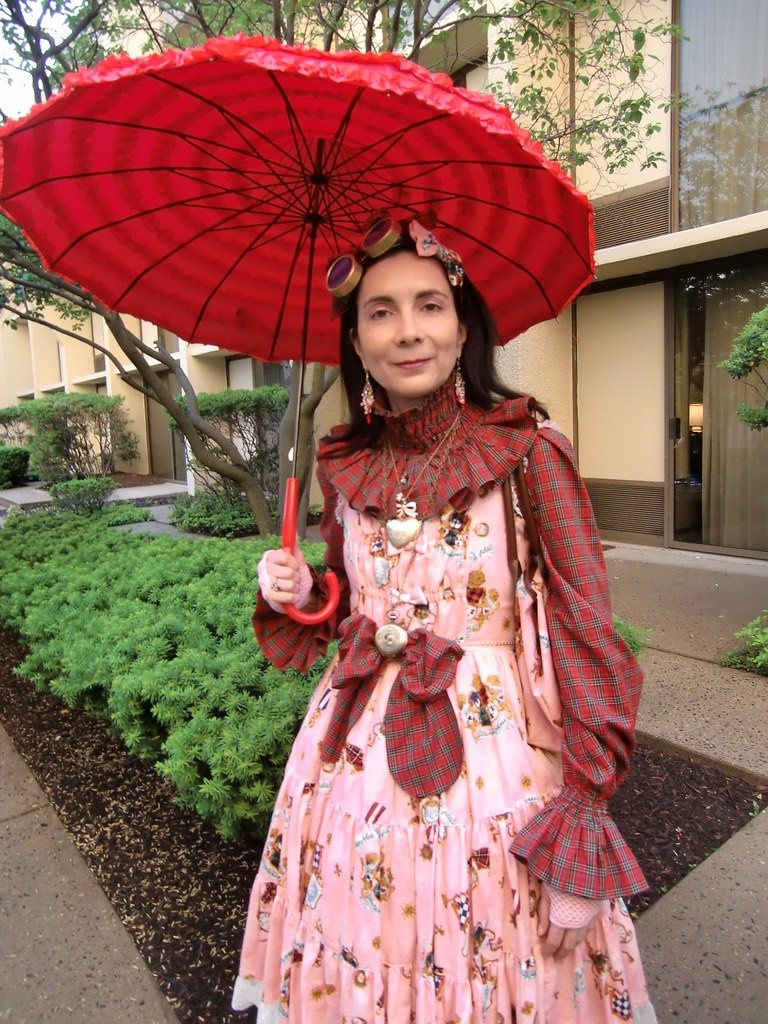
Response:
[231,218,656,1024]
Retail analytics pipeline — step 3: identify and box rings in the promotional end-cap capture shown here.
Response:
[271,578,281,592]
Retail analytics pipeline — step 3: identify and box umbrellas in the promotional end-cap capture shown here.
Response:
[0,32,598,625]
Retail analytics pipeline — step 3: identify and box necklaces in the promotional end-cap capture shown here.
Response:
[374,412,462,658]
